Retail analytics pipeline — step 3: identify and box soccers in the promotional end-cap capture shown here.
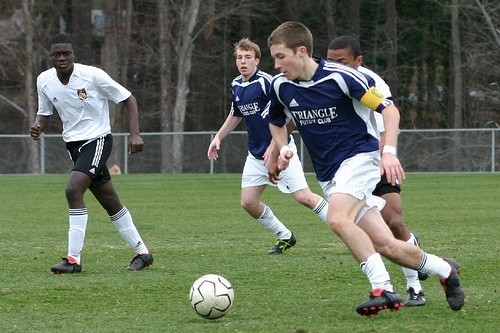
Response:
[189,274,234,319]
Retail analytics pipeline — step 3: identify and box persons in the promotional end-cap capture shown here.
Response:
[207,39,329,254]
[268,22,466,318]
[326,36,429,308]
[29,33,154,274]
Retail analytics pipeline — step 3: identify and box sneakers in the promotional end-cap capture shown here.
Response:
[404,287,425,306]
[268,231,296,254]
[356,288,404,318]
[410,231,420,248]
[50,257,82,274]
[127,252,154,270]
[439,257,465,311]
[418,271,428,281]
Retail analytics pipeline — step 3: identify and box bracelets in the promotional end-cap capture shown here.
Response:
[280,146,291,154]
[383,144,396,155]
